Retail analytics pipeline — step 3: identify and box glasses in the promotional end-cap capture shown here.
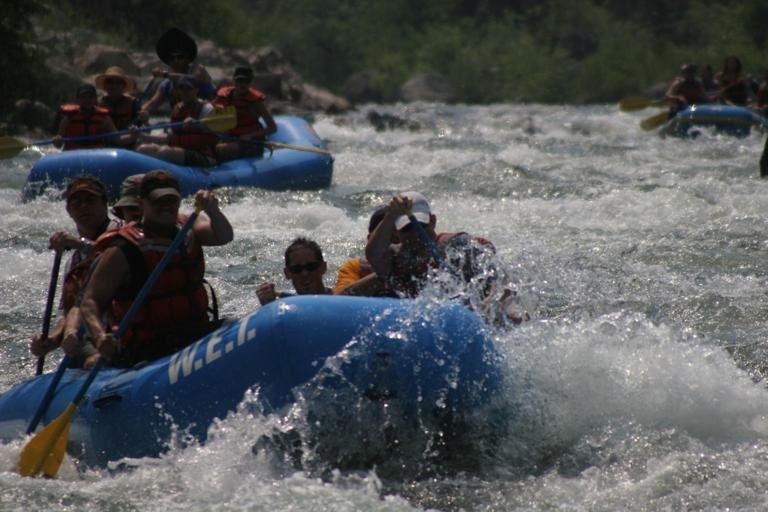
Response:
[288,260,320,273]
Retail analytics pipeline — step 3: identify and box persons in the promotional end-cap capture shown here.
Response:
[30,173,122,359]
[126,28,218,126]
[133,75,220,167]
[663,53,767,121]
[61,172,146,370]
[363,191,532,334]
[52,82,141,154]
[85,67,149,151]
[197,64,278,161]
[255,234,333,305]
[79,170,235,373]
[332,204,396,296]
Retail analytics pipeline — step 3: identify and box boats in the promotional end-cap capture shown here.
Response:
[0,294,504,471]
[18,114,333,204]
[657,105,766,143]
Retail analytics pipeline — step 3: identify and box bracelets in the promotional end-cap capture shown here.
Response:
[79,236,92,255]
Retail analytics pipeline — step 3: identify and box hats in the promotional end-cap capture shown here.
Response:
[75,28,253,98]
[394,191,431,231]
[66,169,182,210]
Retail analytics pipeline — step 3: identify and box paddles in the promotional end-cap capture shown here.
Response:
[20,204,203,477]
[641,103,679,131]
[620,93,671,112]
[1,107,236,163]
[213,133,336,157]
